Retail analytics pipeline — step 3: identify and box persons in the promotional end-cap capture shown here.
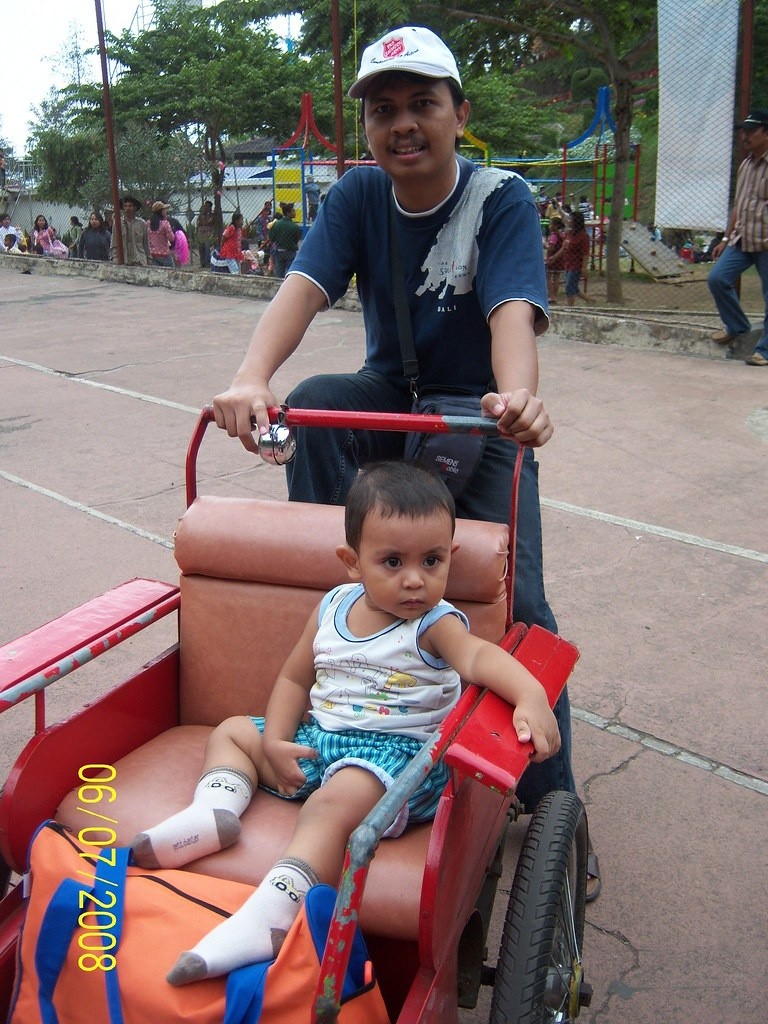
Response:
[668,229,700,253]
[62,216,83,253]
[578,195,594,236]
[214,24,600,902]
[542,217,564,304]
[5,234,21,253]
[110,195,188,268]
[270,204,302,278]
[0,210,112,260]
[197,201,282,274]
[704,230,724,259]
[536,185,577,242]
[707,109,768,367]
[130,461,563,986]
[547,212,595,307]
[304,175,320,222]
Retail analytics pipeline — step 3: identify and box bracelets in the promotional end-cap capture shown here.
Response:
[722,237,729,242]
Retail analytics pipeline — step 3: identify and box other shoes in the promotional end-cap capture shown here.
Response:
[711,329,733,344]
[745,352,768,366]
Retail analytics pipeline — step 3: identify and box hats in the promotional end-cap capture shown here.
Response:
[734,112,768,129]
[348,25,463,97]
[119,195,141,211]
[151,200,170,213]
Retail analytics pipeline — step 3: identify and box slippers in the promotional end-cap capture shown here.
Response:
[585,838,600,902]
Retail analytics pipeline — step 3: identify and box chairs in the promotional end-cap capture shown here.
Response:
[40,490,511,943]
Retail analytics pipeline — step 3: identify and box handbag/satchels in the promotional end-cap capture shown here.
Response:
[405,396,488,500]
[46,230,68,258]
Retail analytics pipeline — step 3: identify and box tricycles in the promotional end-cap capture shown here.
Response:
[0,399,603,1023]
